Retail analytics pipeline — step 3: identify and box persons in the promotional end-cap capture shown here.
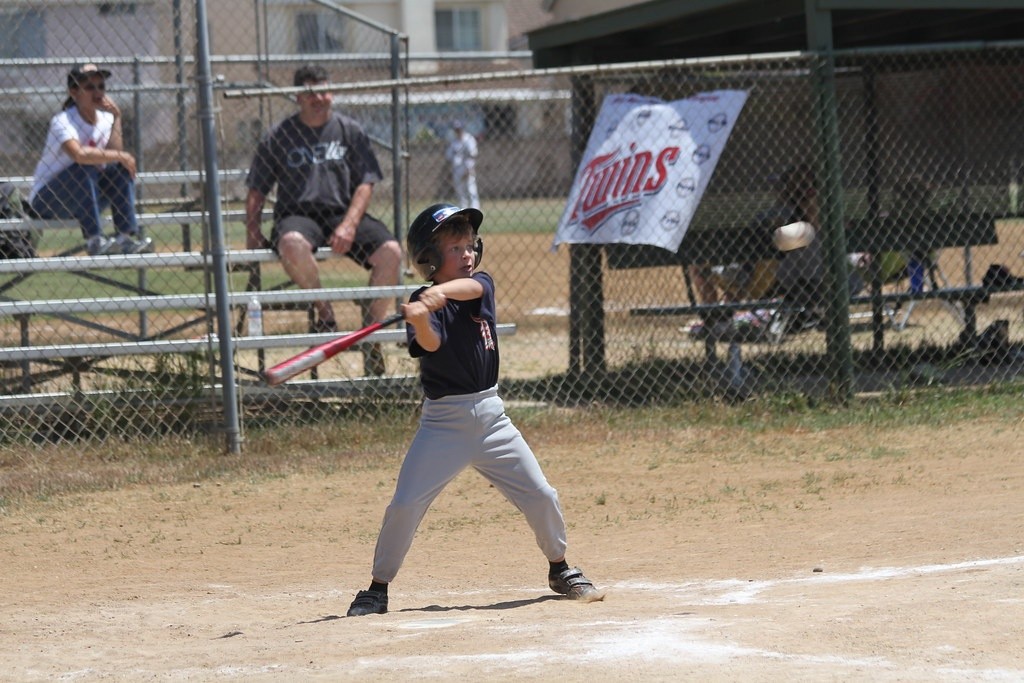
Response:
[247,66,402,335]
[444,120,480,209]
[686,259,782,340]
[28,63,156,254]
[347,205,604,617]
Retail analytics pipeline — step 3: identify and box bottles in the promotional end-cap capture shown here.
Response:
[247,297,262,336]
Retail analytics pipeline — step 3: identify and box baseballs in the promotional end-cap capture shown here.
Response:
[773,225,800,252]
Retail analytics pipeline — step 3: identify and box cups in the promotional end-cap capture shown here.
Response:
[908,263,923,293]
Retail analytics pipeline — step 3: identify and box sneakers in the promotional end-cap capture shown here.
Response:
[346,590,389,616]
[547,567,605,601]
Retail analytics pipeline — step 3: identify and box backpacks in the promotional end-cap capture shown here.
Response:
[0,182,43,260]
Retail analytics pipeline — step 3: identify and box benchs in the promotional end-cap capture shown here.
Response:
[628,282,990,362]
[0,168,515,415]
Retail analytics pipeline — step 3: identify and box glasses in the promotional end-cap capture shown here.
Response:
[82,83,106,91]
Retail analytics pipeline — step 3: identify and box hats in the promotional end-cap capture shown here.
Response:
[67,63,112,85]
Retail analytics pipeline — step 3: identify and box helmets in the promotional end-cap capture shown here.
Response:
[406,203,483,282]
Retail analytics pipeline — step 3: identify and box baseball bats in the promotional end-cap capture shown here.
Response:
[265,293,448,386]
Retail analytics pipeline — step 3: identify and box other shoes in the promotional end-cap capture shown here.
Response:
[309,319,339,333]
[83,235,122,255]
[361,343,386,377]
[117,232,156,253]
